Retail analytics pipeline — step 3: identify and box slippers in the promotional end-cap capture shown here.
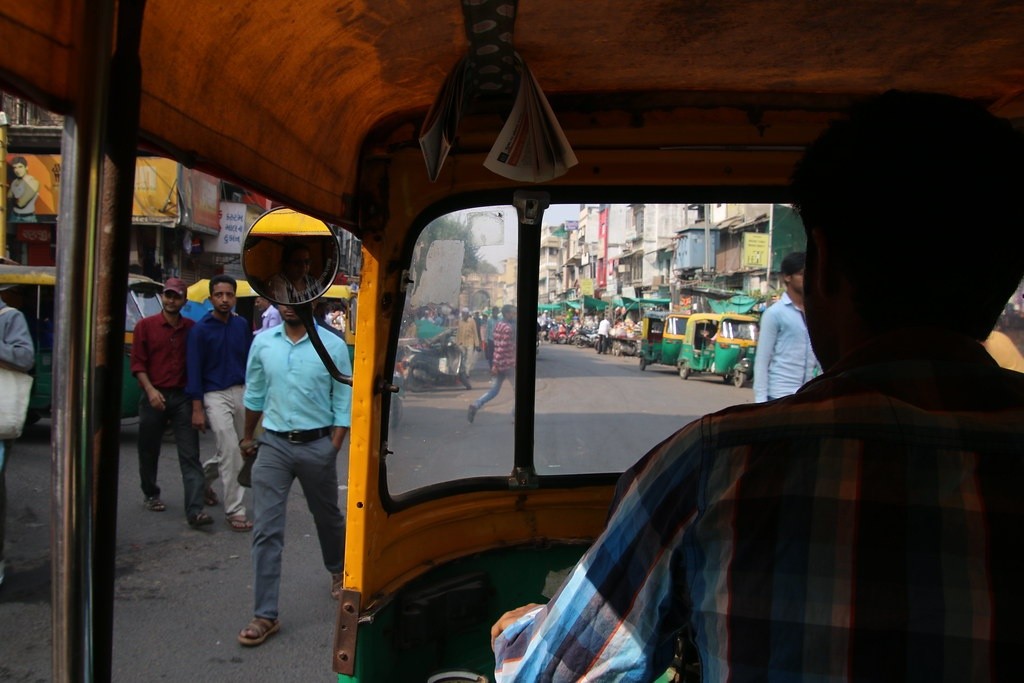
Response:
[225,515,252,534]
[207,490,217,507]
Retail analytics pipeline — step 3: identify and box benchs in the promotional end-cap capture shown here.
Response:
[649,333,662,351]
[695,335,714,358]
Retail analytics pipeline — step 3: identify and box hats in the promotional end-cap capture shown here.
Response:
[163,278,188,299]
[461,307,468,312]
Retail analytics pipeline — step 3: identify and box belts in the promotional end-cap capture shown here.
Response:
[262,427,334,445]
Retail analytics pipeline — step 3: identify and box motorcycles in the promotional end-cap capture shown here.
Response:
[0,262,360,429]
[638,307,759,388]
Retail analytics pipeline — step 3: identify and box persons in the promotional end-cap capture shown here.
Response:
[265,243,322,303]
[325,302,347,332]
[573,313,579,325]
[5,155,39,222]
[538,310,549,341]
[468,304,516,423]
[186,275,253,531]
[598,318,610,354]
[561,320,567,328]
[753,252,823,404]
[0,299,35,582]
[237,301,352,646]
[415,302,499,378]
[252,296,282,335]
[489,89,1023,683]
[130,278,215,525]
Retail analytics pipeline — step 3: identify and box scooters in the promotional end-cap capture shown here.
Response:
[547,318,598,347]
[403,325,474,393]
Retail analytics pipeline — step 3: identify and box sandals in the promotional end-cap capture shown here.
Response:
[330,575,341,599]
[237,615,284,646]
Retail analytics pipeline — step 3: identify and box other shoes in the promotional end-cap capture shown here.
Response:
[466,405,475,426]
[186,511,214,527]
[143,499,166,512]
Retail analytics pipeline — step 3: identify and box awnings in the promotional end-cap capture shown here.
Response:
[562,296,609,312]
[537,305,561,317]
[612,298,671,311]
[704,294,761,314]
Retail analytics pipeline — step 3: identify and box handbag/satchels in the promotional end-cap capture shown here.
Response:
[1,371,33,440]
[237,443,259,490]
